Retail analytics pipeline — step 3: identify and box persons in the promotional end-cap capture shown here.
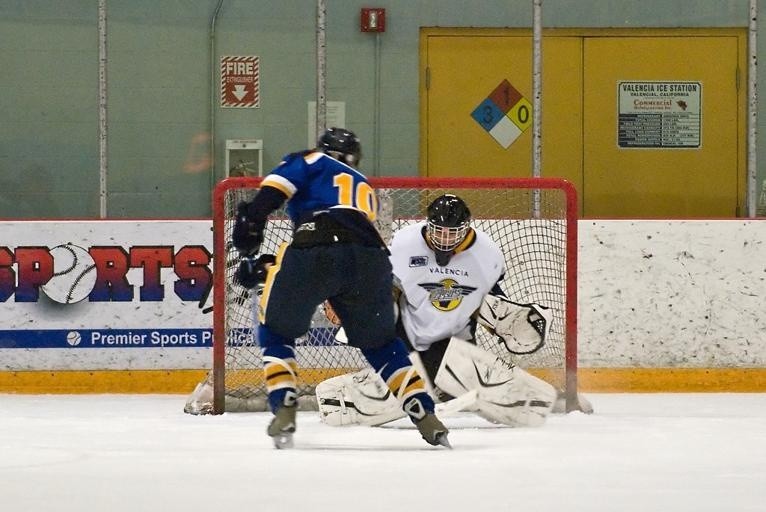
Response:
[314,194,552,424]
[234,128,449,449]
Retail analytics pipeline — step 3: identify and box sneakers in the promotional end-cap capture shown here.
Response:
[267,400,299,437]
[413,410,449,446]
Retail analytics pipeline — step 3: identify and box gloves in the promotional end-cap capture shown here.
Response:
[231,201,276,289]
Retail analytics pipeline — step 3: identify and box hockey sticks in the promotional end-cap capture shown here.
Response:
[394,300,479,417]
[248,256,311,346]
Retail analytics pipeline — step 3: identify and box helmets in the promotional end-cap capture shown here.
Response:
[316,126,361,170]
[426,195,471,252]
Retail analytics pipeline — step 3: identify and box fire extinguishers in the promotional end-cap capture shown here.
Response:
[229,159,255,218]
